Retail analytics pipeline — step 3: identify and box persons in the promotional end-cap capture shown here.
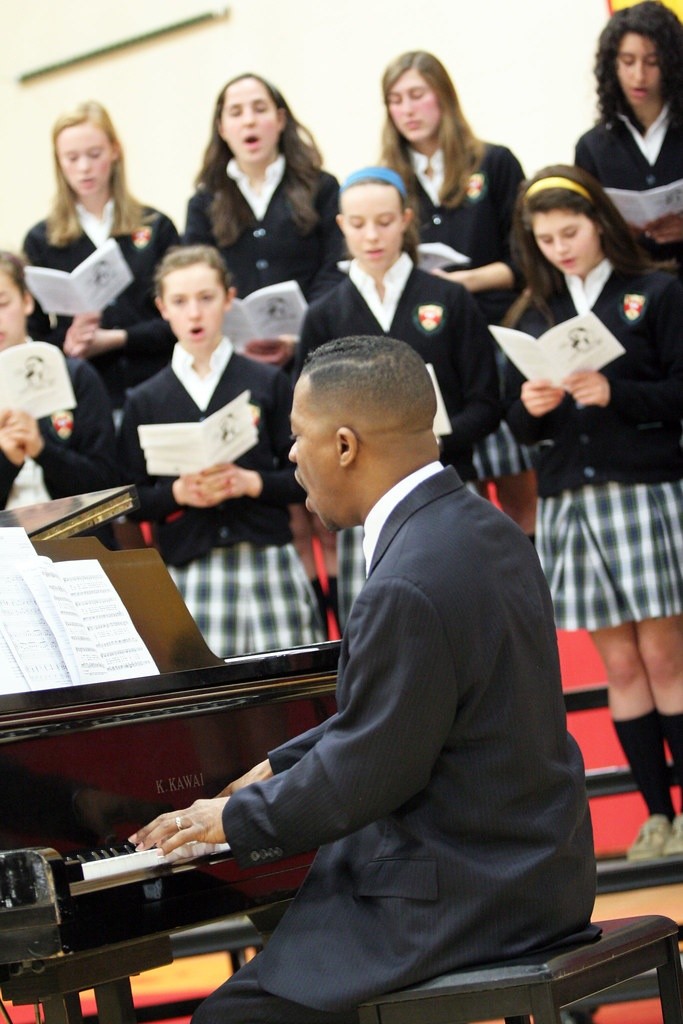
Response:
[1,251,124,553]
[116,242,326,659]
[501,168,681,868]
[373,50,536,513]
[19,97,185,429]
[295,167,502,502]
[182,71,349,644]
[126,337,597,1024]
[574,0,683,290]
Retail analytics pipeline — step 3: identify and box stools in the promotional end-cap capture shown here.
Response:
[357,915,683,1024]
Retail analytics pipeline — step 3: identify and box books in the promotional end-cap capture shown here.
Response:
[2,306,633,479]
[21,234,136,320]
[602,178,683,227]
[220,280,310,355]
[334,239,469,279]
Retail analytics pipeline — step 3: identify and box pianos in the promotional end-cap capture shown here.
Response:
[2,482,344,1024]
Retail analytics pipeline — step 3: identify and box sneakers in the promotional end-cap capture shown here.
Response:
[627,814,683,862]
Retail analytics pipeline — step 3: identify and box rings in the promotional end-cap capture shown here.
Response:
[176,816,184,831]
